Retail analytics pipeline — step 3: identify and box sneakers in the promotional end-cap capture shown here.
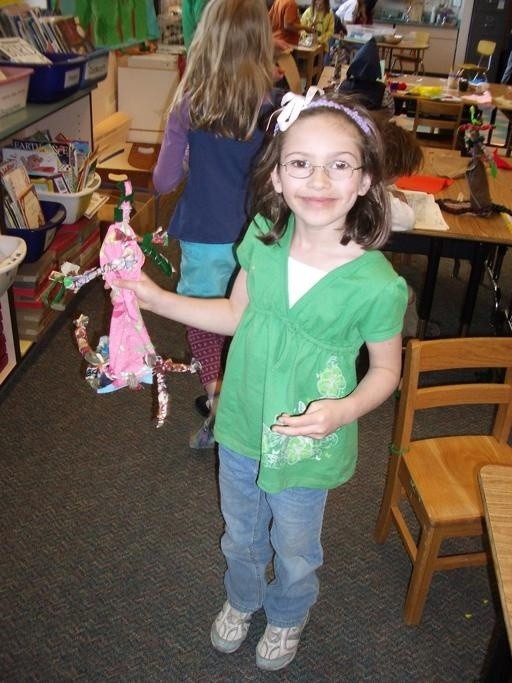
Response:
[194,395,211,417]
[207,598,253,656]
[255,609,311,672]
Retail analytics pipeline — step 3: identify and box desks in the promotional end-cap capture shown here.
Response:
[475,461,512,655]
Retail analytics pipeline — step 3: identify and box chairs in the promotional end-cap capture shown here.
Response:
[375,330,512,629]
[285,16,511,411]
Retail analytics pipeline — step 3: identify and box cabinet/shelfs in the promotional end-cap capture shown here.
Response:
[1,82,113,403]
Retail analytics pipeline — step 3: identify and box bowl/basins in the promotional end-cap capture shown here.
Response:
[383,34,405,45]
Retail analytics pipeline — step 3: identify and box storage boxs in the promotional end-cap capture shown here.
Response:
[80,44,112,90]
[0,63,38,119]
[90,110,135,162]
[1,50,90,105]
[96,185,158,244]
[1,234,32,296]
[31,168,103,226]
[1,198,68,264]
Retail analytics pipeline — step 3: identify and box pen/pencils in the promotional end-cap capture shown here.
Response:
[98,148,126,163]
[449,64,465,75]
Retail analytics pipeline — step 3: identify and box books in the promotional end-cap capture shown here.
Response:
[0,144,102,344]
[0,4,96,64]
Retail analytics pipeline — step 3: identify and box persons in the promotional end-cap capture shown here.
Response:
[333,37,395,116]
[181,0,208,56]
[299,0,334,95]
[268,0,315,94]
[104,94,412,672]
[150,0,285,452]
[335,0,362,25]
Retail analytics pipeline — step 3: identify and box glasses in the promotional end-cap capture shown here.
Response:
[279,155,364,182]
